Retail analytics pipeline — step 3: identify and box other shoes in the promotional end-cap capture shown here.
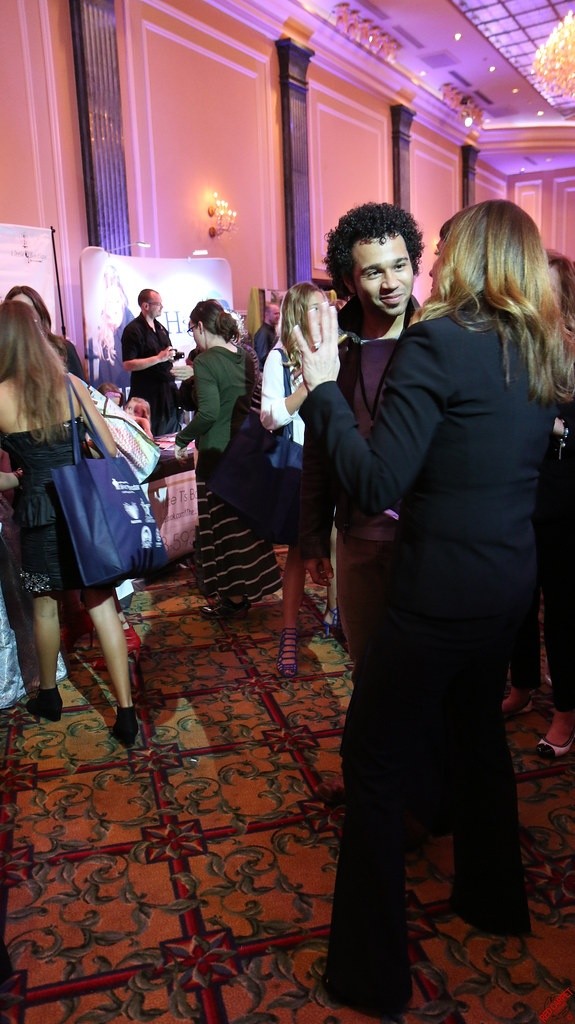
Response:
[501,698,533,718]
[536,724,575,759]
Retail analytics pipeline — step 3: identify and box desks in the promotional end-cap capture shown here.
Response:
[56,432,199,653]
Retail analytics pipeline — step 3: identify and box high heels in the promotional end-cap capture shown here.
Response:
[111,706,138,748]
[27,685,63,722]
[322,606,345,637]
[276,627,298,677]
[91,624,141,669]
[201,598,252,618]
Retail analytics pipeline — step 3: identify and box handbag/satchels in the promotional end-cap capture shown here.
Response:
[207,349,305,544]
[51,374,170,586]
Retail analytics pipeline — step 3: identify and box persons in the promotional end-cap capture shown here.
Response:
[503,255,574,757]
[121,288,183,435]
[325,203,423,662]
[102,382,154,441]
[1,301,138,748]
[293,200,575,1024]
[175,284,349,676]
[1,284,139,651]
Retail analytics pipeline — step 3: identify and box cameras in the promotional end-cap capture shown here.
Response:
[170,349,186,362]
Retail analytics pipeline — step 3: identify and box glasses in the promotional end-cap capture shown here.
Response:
[187,325,197,337]
[149,302,162,307]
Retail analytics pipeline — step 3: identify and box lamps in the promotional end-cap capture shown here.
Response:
[208,192,236,240]
[531,9,575,99]
[186,250,209,259]
[106,241,150,252]
